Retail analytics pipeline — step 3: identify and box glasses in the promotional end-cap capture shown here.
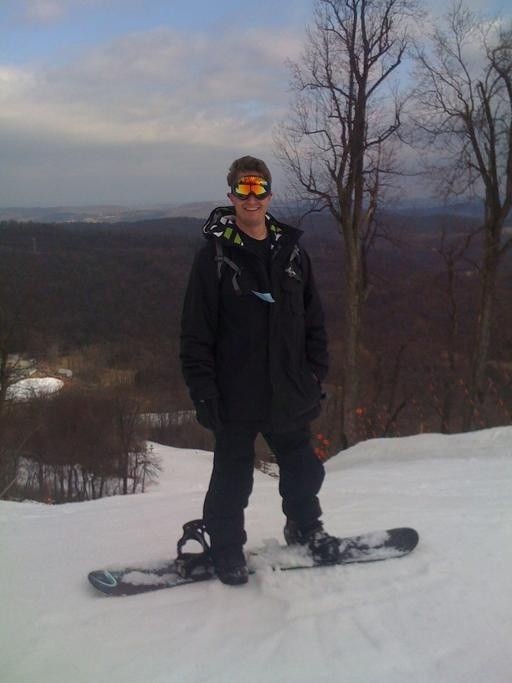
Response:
[231,175,271,200]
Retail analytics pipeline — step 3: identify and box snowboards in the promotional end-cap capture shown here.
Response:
[88,520,418,596]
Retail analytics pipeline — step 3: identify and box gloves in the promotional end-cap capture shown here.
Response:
[194,398,227,432]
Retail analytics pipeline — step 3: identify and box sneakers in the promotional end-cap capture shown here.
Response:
[284,515,339,563]
[205,526,248,585]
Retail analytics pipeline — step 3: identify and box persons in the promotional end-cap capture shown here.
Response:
[183,155,341,585]
